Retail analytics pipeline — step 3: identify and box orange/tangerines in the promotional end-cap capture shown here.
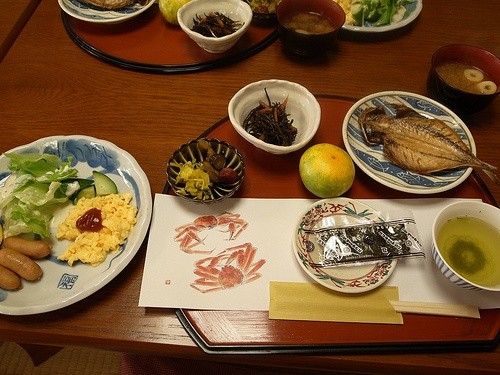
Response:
[158,0,192,25]
[299,143,355,199]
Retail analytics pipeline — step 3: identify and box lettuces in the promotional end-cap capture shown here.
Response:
[350,0,413,27]
[0,152,78,244]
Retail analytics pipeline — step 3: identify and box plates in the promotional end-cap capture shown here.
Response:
[0,135,153,315]
[332,0,423,32]
[341,90,476,194]
[292,197,398,293]
[57,0,155,24]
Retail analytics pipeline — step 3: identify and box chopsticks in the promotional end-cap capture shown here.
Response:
[389,301,481,319]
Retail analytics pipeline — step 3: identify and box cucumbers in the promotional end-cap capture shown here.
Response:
[59,170,118,206]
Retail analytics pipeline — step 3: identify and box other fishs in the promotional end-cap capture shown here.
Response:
[357,103,499,184]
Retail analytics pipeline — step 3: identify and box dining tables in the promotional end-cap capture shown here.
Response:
[0,0,500,375]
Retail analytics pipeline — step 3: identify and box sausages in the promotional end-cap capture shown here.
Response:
[0,236,51,290]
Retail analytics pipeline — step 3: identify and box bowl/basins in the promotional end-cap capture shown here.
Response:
[276,0,345,56]
[165,137,245,203]
[177,0,253,53]
[426,44,500,115]
[227,79,322,155]
[431,201,500,294]
[242,0,279,23]
